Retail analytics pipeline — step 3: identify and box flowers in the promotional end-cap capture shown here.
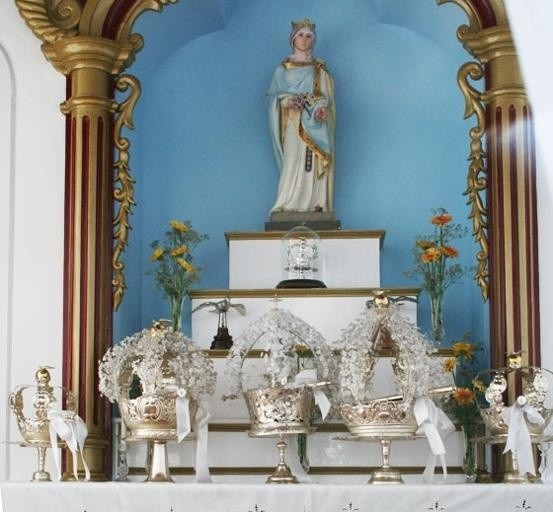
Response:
[296,344,308,373]
[441,336,489,470]
[402,208,477,329]
[144,219,209,331]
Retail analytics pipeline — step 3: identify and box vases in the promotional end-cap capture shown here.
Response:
[169,293,186,332]
[461,415,477,483]
[427,293,446,344]
[296,434,310,474]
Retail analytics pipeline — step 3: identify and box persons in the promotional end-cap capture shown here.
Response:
[263,18,338,214]
